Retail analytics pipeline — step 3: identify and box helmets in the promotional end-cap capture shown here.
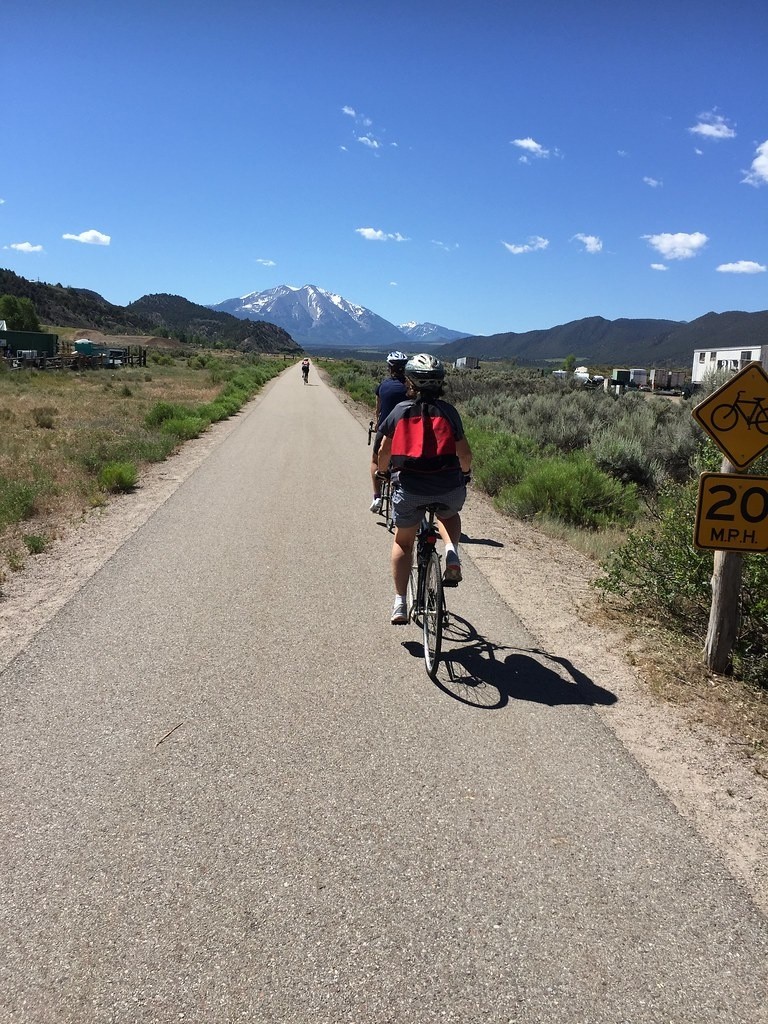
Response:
[386,351,409,367]
[304,357,308,361]
[402,353,445,387]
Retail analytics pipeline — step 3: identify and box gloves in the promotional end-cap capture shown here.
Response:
[374,469,391,485]
[461,468,472,485]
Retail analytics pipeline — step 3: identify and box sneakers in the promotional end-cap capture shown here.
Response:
[390,603,407,621]
[442,550,463,582]
[369,498,382,513]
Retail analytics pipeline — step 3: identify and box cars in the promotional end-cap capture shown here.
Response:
[638,382,652,393]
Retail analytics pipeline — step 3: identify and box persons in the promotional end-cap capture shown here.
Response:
[374,353,473,624]
[302,359,310,383]
[369,350,408,512]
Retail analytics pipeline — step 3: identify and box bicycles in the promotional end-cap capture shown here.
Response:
[302,371,307,385]
[367,420,401,532]
[383,465,459,679]
